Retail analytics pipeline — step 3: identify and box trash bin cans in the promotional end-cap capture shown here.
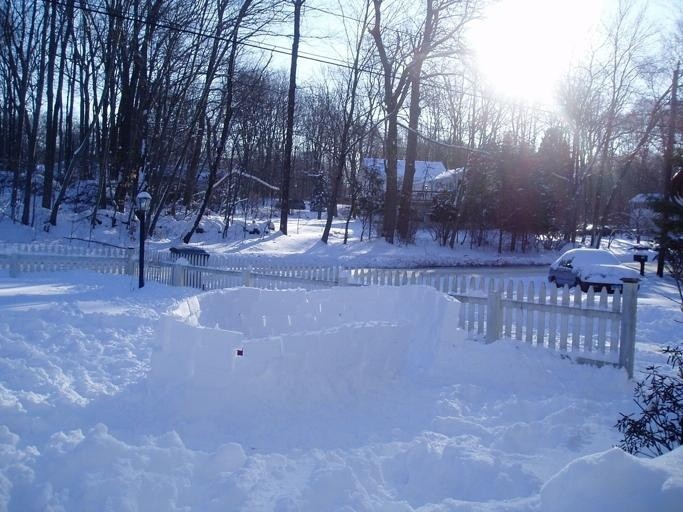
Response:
[169,245,210,287]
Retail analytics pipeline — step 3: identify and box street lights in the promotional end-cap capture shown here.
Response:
[134,190,157,288]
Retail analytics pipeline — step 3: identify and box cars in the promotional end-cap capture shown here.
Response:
[548,248,641,294]
